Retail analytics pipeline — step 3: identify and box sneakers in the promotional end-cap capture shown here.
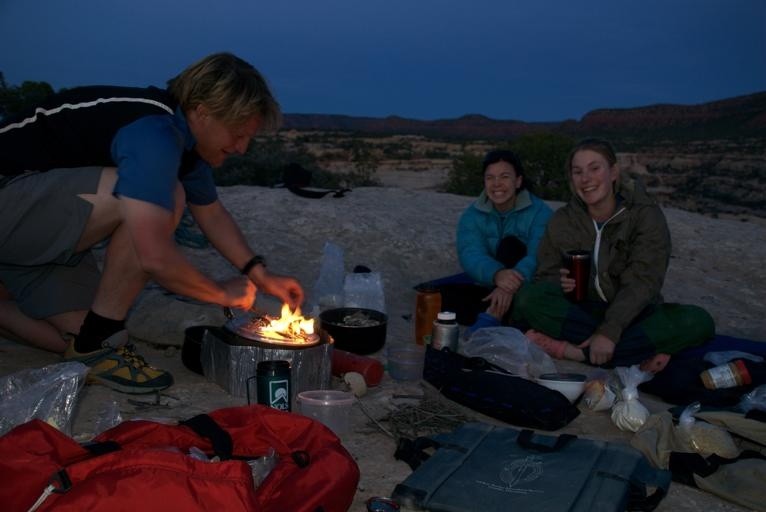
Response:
[61,330,174,395]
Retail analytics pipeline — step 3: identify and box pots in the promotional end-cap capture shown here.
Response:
[318,307,389,356]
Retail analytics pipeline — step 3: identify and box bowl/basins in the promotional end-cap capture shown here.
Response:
[535,378,587,406]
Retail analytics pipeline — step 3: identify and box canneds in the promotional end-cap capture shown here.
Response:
[699,359,752,390]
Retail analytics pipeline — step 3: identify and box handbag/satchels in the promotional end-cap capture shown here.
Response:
[393,421,647,512]
[423,345,581,429]
[0,404,361,512]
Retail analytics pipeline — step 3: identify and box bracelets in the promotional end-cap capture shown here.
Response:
[240,253,267,276]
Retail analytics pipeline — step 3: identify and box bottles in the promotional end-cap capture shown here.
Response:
[256,360,293,413]
[429,311,459,354]
[567,248,590,302]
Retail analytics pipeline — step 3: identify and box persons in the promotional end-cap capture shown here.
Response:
[422,149,554,341]
[511,137,716,374]
[0,50,306,397]
